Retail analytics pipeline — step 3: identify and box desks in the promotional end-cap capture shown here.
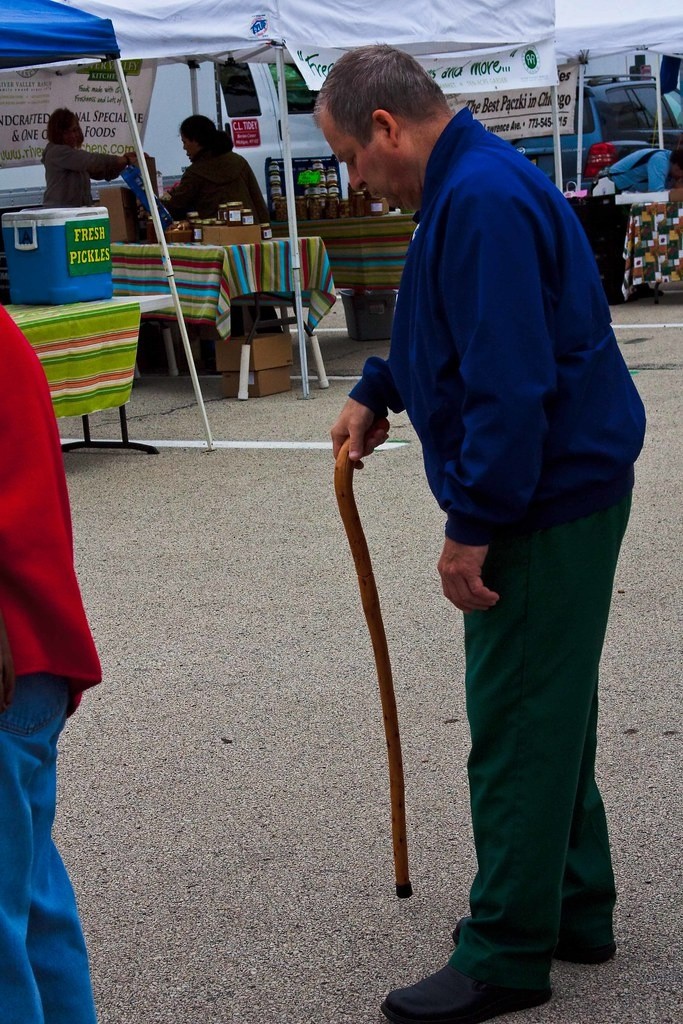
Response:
[621,200,683,304]
[108,232,347,398]
[11,289,179,459]
[270,211,420,336]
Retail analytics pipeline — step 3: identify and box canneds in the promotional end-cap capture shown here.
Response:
[161,155,387,244]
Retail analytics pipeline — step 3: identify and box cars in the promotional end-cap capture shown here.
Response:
[0,55,683,212]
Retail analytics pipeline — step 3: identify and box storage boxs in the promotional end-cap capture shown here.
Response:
[217,334,296,398]
[2,204,116,307]
[340,283,400,343]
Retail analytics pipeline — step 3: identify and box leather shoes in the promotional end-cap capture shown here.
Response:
[381,916,616,1024]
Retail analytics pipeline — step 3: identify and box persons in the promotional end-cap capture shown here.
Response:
[163,115,270,227]
[311,45,644,1024]
[41,106,147,209]
[0,301,102,1022]
[598,147,683,297]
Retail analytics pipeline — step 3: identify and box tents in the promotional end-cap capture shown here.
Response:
[1,2,216,453]
[62,2,564,391]
[556,2,683,197]
